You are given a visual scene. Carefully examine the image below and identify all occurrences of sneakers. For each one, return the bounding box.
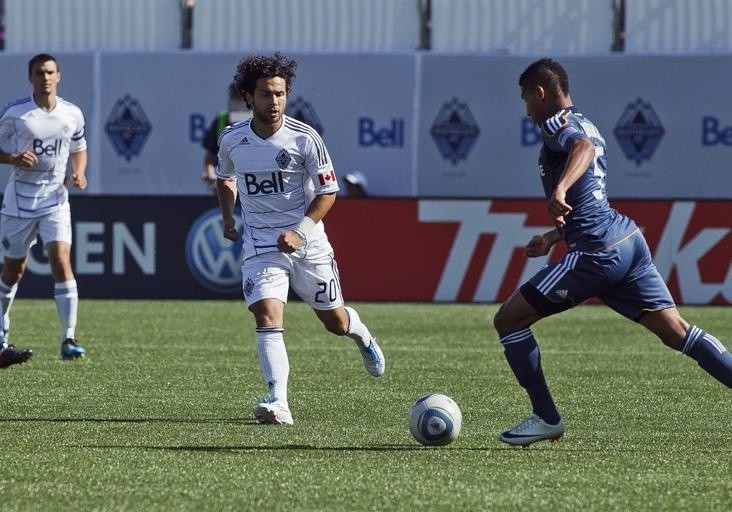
[61,338,85,360]
[359,333,385,377]
[499,414,564,448]
[254,401,293,425]
[0,342,33,368]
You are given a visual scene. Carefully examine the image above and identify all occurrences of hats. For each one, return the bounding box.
[345,171,368,190]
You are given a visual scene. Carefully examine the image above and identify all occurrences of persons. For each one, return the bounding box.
[203,83,253,189]
[0,301,33,368]
[0,53,87,358]
[214,53,386,425]
[494,59,732,445]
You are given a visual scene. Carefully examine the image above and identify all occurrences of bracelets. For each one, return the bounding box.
[292,215,316,239]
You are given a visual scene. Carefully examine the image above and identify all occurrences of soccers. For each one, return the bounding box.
[407,393,462,446]
